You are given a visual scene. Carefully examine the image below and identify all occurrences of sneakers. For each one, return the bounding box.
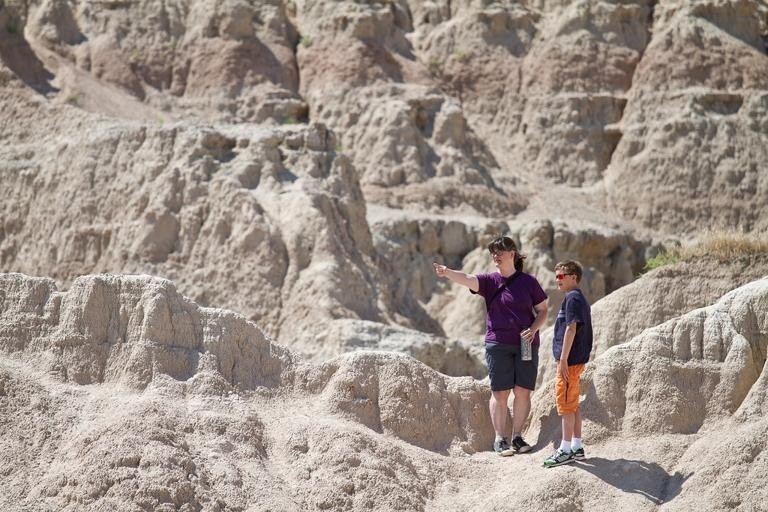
[543,447,575,468]
[511,435,532,454]
[493,438,514,457]
[571,447,585,461]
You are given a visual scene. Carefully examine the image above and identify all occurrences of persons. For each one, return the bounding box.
[433,236,548,456]
[543,260,593,467]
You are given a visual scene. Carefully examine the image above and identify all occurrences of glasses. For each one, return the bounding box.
[555,273,572,279]
[490,250,513,257]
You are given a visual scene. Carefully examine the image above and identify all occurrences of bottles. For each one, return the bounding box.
[520,327,532,361]
